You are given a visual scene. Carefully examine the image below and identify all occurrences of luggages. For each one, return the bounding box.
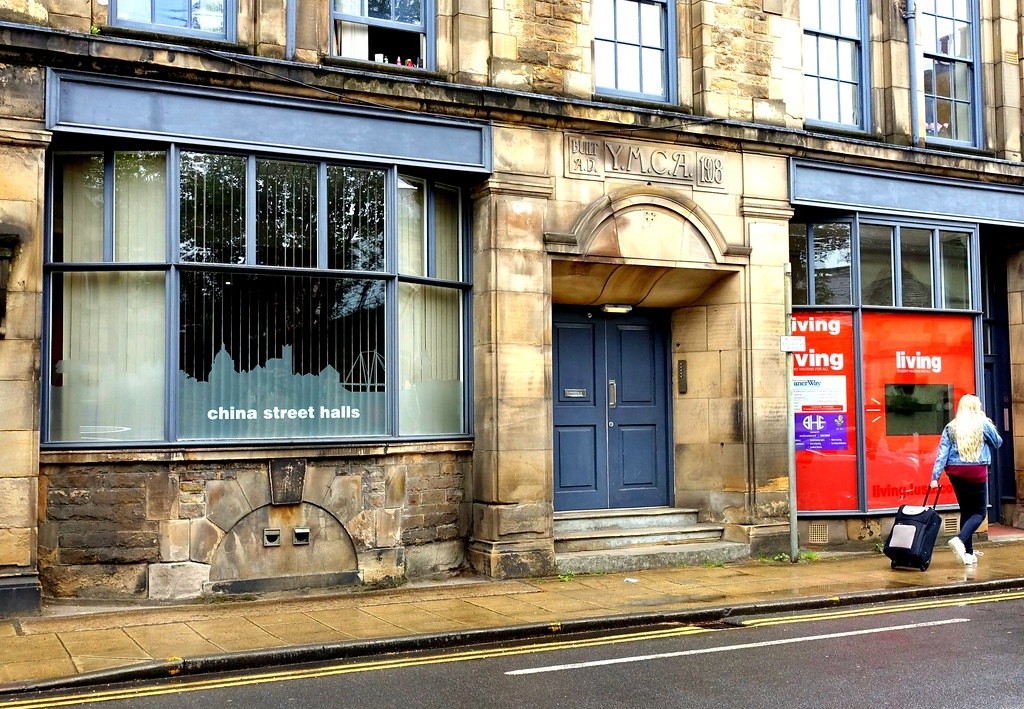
[883,483,942,572]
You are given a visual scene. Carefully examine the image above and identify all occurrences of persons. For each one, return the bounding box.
[928,393,1003,566]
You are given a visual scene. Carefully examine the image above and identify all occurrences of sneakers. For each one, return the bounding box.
[948,536,985,565]
